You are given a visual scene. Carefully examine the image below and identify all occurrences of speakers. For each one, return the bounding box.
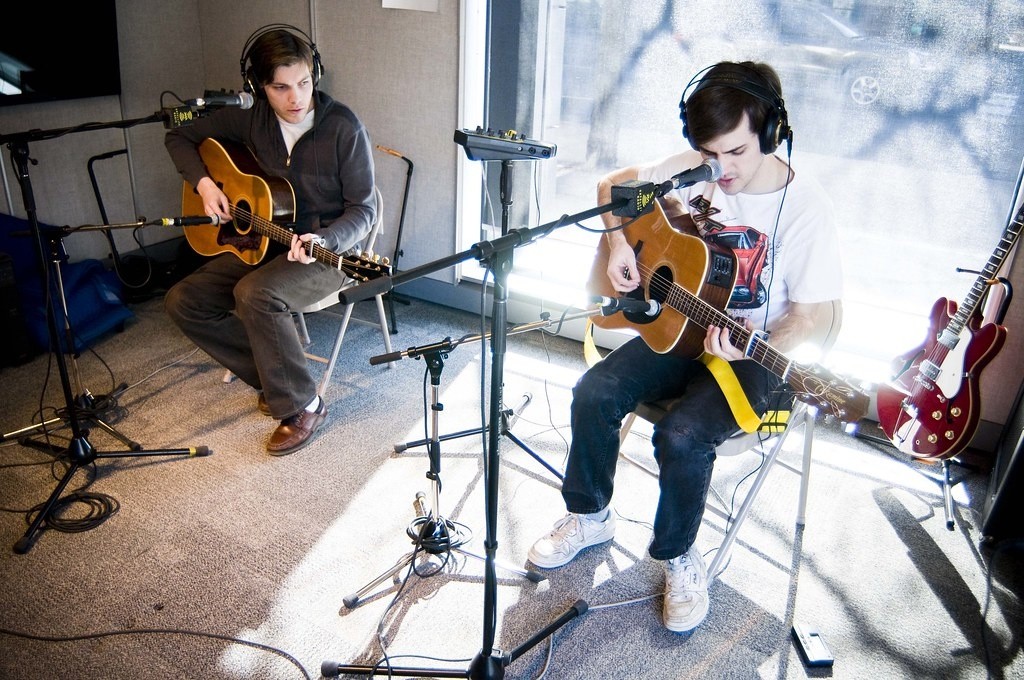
[979,377,1024,539]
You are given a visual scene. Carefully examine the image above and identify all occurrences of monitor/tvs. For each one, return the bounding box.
[0,0,121,107]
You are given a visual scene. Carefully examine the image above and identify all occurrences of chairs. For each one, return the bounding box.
[221,185,396,399]
[623,296,842,586]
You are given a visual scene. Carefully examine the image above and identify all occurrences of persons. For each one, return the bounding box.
[163,30,376,455]
[527,61,845,631]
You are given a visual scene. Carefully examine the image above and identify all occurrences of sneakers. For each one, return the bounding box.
[658,542,709,631]
[528,507,617,569]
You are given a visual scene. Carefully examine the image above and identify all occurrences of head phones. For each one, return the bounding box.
[239,22,325,101]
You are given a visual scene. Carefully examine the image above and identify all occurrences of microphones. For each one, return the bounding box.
[183,91,254,110]
[591,294,662,317]
[154,212,221,228]
[653,158,724,198]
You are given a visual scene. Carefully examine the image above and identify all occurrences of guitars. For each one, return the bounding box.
[584,193,870,424]
[876,204,1023,462]
[180,136,392,284]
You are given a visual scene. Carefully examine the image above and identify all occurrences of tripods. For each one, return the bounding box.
[847,156,1024,528]
[0,145,208,554]
[321,160,590,680]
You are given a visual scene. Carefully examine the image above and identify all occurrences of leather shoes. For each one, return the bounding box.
[257,391,271,416]
[265,394,330,457]
